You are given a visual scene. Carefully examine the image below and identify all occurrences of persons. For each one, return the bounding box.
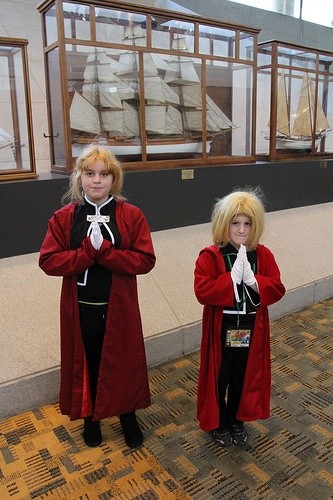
[38,148,156,448]
[193,191,286,445]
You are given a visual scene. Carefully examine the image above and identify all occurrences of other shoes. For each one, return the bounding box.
[209,427,232,446]
[231,419,248,446]
[83,415,102,447]
[120,412,145,448]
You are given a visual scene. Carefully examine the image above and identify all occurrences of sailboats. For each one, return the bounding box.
[264,67,332,154]
[70,24,239,161]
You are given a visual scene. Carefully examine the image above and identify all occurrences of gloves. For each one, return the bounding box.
[230,243,243,285]
[90,222,98,250]
[243,245,256,286]
[95,221,104,251]
